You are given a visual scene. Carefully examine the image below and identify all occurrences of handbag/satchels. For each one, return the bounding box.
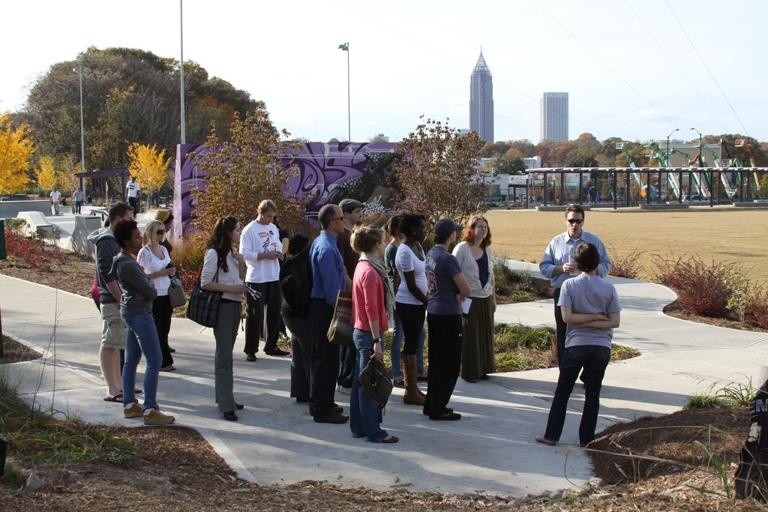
[327,288,354,346]
[359,357,392,407]
[167,263,187,308]
[186,248,223,327]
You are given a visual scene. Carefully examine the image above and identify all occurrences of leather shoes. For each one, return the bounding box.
[224,412,238,422]
[444,407,453,412]
[536,436,556,445]
[265,347,290,355]
[236,402,243,409]
[313,413,350,423]
[308,406,343,415]
[246,353,256,361]
[369,434,400,444]
[429,411,461,420]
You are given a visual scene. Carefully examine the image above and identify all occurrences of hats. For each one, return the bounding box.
[339,198,366,214]
[433,218,464,244]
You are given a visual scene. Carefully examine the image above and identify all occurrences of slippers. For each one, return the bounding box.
[121,387,142,394]
[104,394,137,403]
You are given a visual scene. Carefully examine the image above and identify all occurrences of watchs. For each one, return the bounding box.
[373,337,382,343]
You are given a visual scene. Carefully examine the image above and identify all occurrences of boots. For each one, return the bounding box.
[402,354,426,405]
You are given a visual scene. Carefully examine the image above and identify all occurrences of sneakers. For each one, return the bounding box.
[143,409,177,425]
[340,386,351,393]
[124,402,143,418]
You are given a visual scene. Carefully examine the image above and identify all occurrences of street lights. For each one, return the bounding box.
[333,41,350,141]
[73,64,86,198]
[666,129,680,202]
[688,125,702,202]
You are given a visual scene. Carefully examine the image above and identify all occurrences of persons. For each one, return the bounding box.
[336,199,366,392]
[539,204,612,388]
[50,187,62,215]
[87,204,143,403]
[395,213,429,405]
[239,199,290,361]
[261,216,288,341]
[125,176,141,219]
[351,226,398,444]
[143,209,175,352]
[73,188,84,214]
[381,215,427,389]
[280,234,310,403]
[423,219,471,421]
[306,205,349,425]
[536,244,622,447]
[107,218,175,425]
[136,220,175,371]
[201,216,247,421]
[452,216,496,383]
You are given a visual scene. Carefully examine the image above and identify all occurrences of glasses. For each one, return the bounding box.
[327,215,344,222]
[568,218,583,224]
[156,229,165,236]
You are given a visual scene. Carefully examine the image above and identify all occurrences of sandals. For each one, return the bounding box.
[158,366,176,371]
